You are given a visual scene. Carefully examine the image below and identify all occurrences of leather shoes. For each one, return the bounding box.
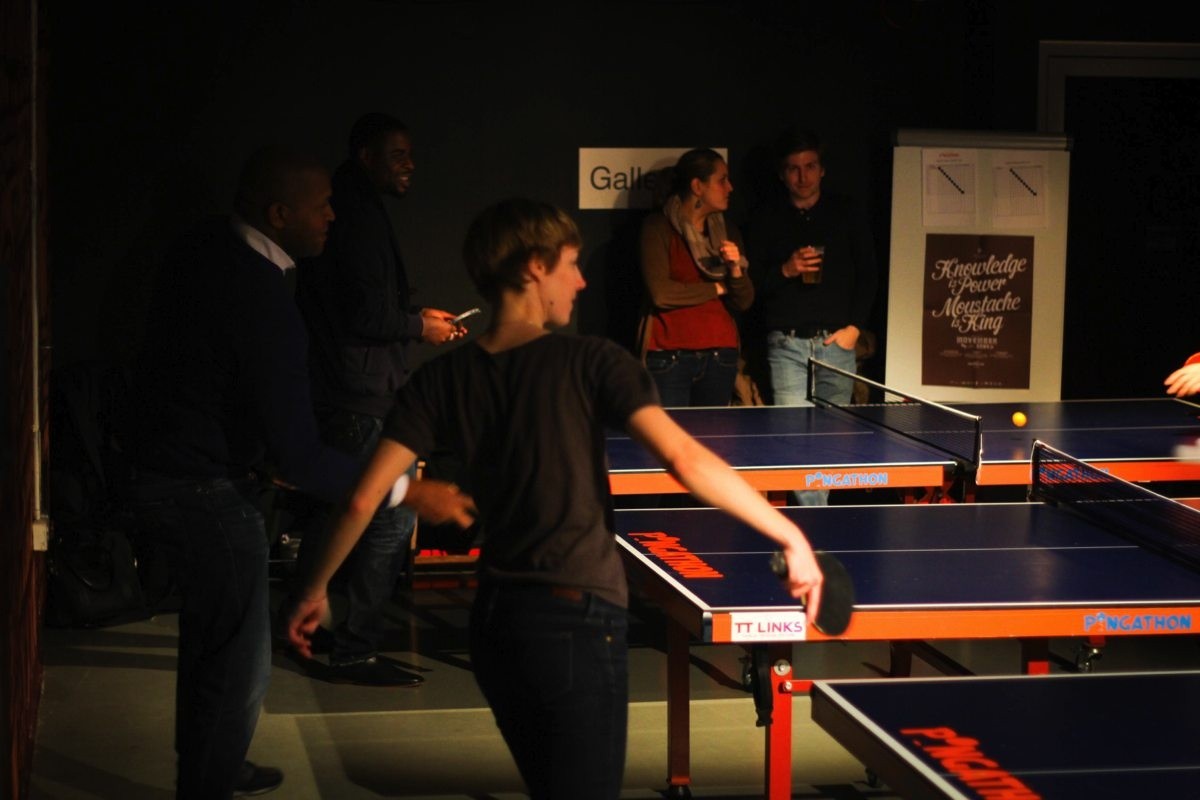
[305,655,423,686]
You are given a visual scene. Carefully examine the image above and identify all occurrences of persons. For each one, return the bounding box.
[294,111,468,686]
[635,148,755,405]
[133,144,480,800]
[744,131,877,406]
[1164,351,1200,398]
[289,198,826,800]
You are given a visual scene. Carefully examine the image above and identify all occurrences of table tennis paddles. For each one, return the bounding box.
[773,551,855,635]
[1183,349,1200,374]
[439,305,483,333]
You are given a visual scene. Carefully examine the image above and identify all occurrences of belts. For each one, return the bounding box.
[783,322,823,339]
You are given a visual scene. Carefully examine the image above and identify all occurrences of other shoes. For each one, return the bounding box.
[227,756,283,797]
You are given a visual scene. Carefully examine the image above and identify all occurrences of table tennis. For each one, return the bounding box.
[1012,411,1028,428]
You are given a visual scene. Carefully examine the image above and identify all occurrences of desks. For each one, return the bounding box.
[602,398,1200,800]
